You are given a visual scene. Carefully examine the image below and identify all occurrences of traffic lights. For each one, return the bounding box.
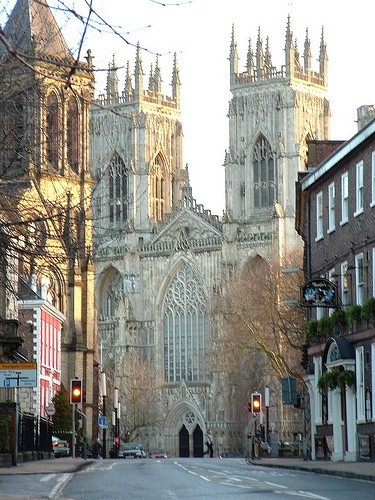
[247,401,252,413]
[250,394,262,414]
[70,379,82,404]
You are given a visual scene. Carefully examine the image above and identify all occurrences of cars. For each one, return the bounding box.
[120,443,147,460]
[51,436,69,457]
[149,449,167,459]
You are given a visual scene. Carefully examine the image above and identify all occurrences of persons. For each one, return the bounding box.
[205,429,214,458]
[253,434,261,458]
[94,437,103,459]
[76,437,86,459]
